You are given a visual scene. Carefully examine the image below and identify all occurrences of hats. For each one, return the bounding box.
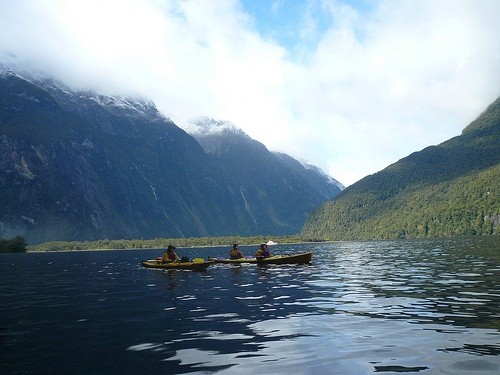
[260,243,267,248]
[166,245,176,250]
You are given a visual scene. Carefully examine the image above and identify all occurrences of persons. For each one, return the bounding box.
[255,244,273,258]
[229,244,245,259]
[162,245,181,264]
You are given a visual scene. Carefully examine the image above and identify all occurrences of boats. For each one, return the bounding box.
[207,252,312,266]
[142,257,216,272]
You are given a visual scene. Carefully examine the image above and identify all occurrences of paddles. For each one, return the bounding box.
[141,257,204,263]
